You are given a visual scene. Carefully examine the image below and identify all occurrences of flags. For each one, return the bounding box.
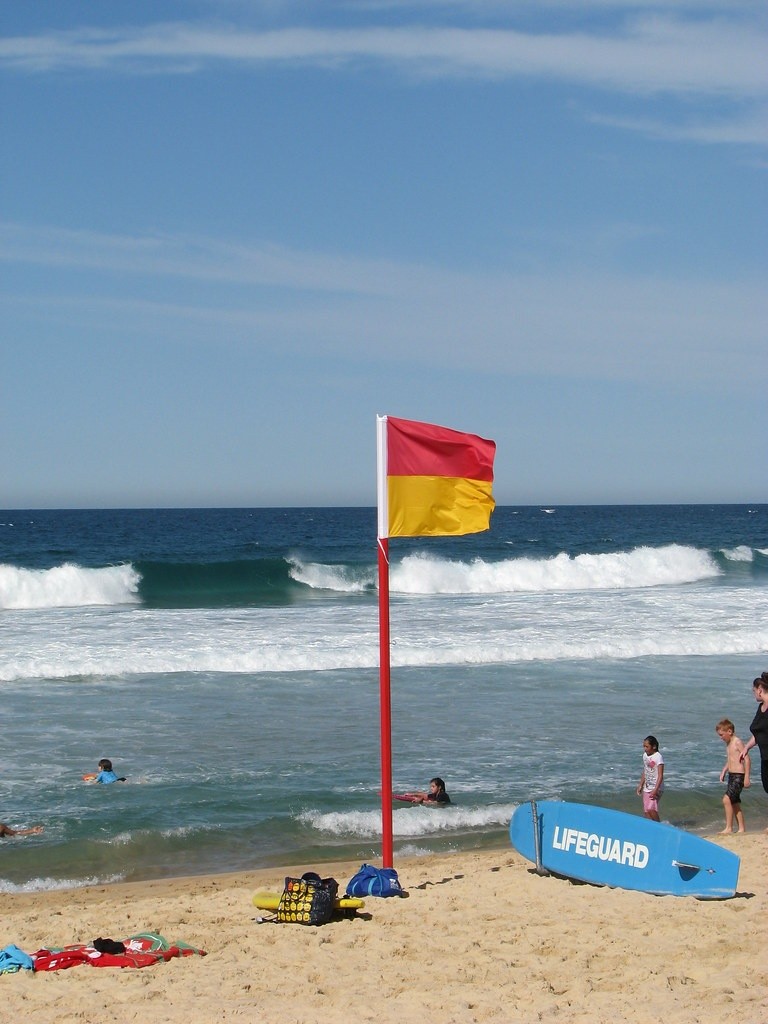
[377,415,496,539]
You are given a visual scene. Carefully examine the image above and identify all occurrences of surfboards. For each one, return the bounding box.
[508,798,743,902]
[376,791,437,805]
[83,772,100,783]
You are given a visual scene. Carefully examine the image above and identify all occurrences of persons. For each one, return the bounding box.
[739,671,768,833]
[716,719,752,834]
[404,778,451,806]
[637,736,665,823]
[92,759,119,785]
[0,822,44,839]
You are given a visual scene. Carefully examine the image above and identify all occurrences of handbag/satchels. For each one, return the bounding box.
[276,877,340,927]
[345,863,402,898]
